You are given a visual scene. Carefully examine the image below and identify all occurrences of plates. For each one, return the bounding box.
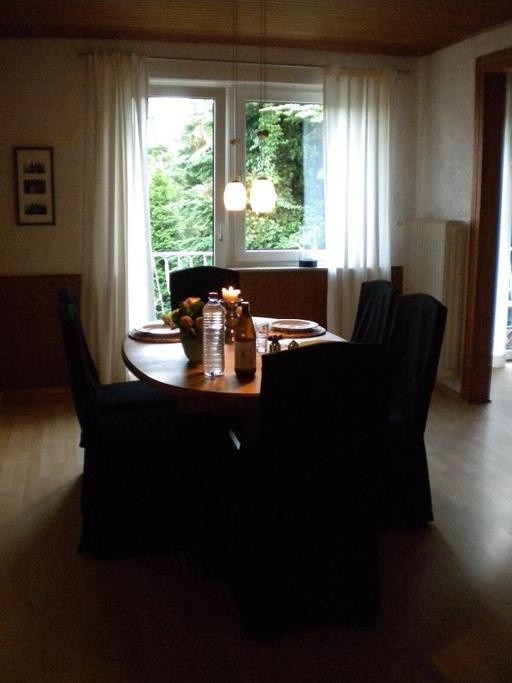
[269,318,319,332]
[134,320,180,336]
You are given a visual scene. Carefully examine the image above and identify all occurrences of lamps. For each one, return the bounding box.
[222,0,277,215]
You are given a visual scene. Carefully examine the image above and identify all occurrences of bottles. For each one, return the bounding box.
[202,291,225,378]
[234,301,257,376]
[222,302,239,345]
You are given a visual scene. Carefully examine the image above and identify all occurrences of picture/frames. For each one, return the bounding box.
[14,144,56,226]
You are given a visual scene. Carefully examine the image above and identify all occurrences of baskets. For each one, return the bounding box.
[180,329,203,363]
